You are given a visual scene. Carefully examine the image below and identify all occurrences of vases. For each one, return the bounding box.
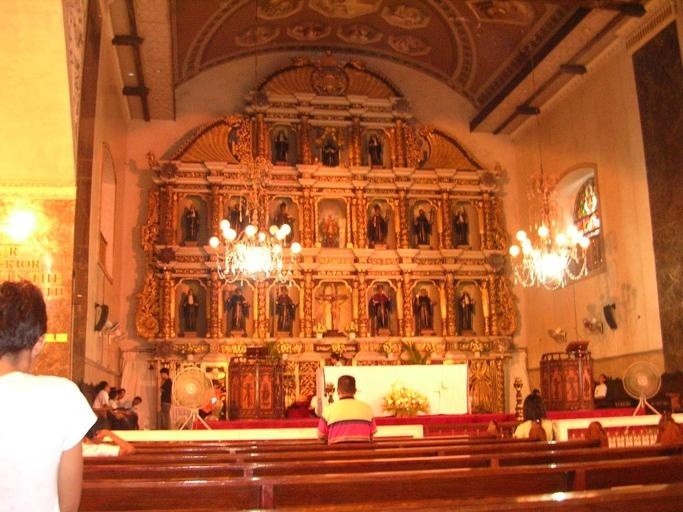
[395,411,417,418]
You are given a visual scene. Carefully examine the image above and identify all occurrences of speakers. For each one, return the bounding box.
[96,304,108,332]
[602,304,618,330]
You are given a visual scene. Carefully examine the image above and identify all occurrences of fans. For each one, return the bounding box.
[622,360,663,416]
[171,367,214,430]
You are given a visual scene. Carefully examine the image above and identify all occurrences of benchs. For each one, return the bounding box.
[75,422,682,512]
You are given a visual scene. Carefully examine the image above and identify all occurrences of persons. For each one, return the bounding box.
[513,393,554,441]
[273,129,288,162]
[159,368,173,431]
[84,377,143,457]
[181,201,469,247]
[182,285,476,331]
[369,135,382,166]
[318,375,377,445]
[322,135,338,167]
[0,278,98,512]
[594,374,608,400]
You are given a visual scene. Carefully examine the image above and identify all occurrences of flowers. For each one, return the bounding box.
[383,386,430,415]
